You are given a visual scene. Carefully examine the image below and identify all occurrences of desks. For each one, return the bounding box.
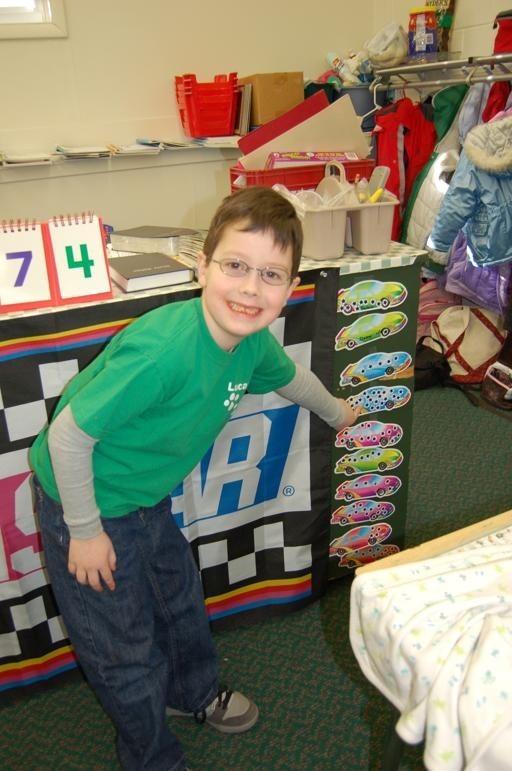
[0,229,429,584]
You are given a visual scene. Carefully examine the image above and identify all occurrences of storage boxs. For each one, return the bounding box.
[238,71,305,128]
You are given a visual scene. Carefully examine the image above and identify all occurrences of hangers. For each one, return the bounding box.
[359,79,412,127]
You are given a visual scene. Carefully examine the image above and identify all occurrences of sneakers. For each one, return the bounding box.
[166,688,259,733]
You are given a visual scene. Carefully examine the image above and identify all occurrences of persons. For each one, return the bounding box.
[28,187,362,771]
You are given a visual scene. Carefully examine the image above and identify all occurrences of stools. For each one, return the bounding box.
[175,69,238,139]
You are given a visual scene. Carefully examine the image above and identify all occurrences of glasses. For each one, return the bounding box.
[207,255,295,286]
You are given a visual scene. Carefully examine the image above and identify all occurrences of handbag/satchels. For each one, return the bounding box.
[414,305,512,411]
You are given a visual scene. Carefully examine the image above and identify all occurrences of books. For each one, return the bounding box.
[107,251,195,293]
[0,134,242,168]
